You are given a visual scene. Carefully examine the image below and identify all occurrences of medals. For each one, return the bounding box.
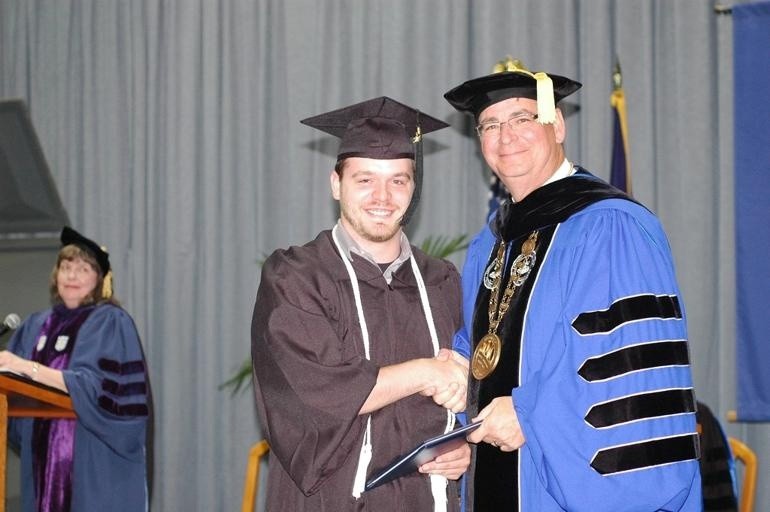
[471,332,502,381]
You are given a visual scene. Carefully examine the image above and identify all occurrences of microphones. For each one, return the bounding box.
[0,311,21,343]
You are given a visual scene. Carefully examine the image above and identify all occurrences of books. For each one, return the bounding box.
[361,418,483,491]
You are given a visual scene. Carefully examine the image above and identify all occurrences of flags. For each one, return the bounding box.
[606,87,633,197]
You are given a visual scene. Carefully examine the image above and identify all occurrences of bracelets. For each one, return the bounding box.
[29,360,40,382]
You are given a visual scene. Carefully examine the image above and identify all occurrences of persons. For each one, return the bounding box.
[0,227,152,510]
[248,97,471,511]
[418,64,705,511]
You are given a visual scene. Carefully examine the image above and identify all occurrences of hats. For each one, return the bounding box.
[444,58,583,124]
[60,226,111,278]
[300,95,451,159]
